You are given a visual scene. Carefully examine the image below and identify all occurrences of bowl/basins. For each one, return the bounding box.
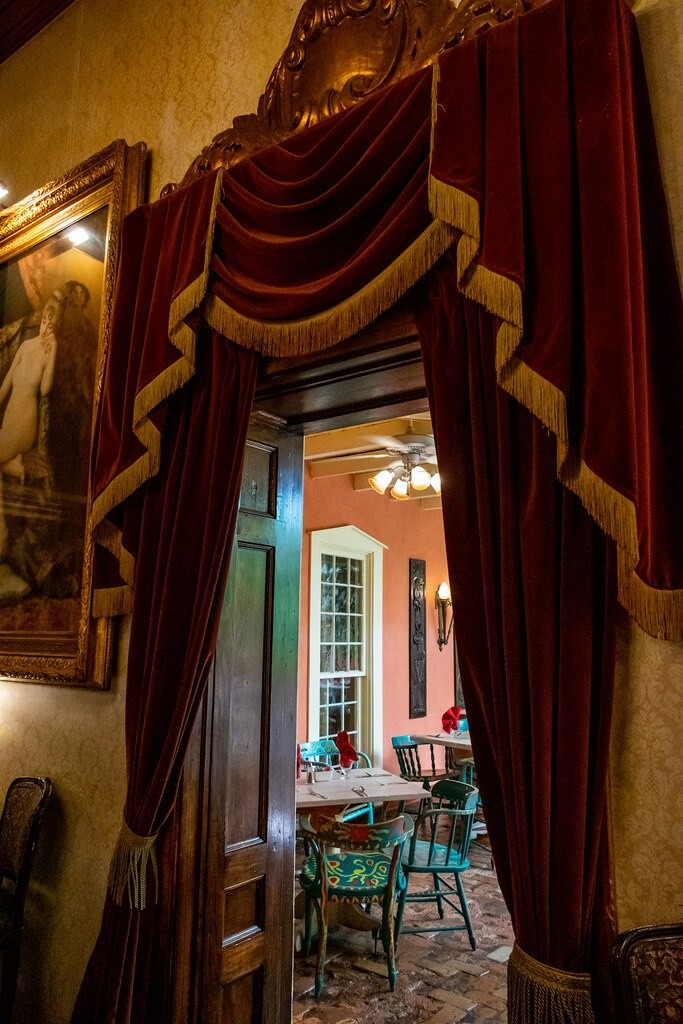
[313,766,334,782]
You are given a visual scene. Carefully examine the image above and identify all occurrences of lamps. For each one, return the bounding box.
[368,419,440,500]
[434,582,453,652]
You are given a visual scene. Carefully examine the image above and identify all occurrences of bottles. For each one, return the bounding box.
[306,761,314,784]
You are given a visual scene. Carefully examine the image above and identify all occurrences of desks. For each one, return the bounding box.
[296,766,432,809]
[409,732,472,750]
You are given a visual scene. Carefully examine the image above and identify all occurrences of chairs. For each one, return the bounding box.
[459,759,496,867]
[451,719,480,802]
[291,815,416,997]
[612,919,683,1024]
[0,776,52,1022]
[305,751,374,825]
[392,736,443,821]
[383,777,479,951]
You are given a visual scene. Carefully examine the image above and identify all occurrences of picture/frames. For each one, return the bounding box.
[1,135,148,693]
